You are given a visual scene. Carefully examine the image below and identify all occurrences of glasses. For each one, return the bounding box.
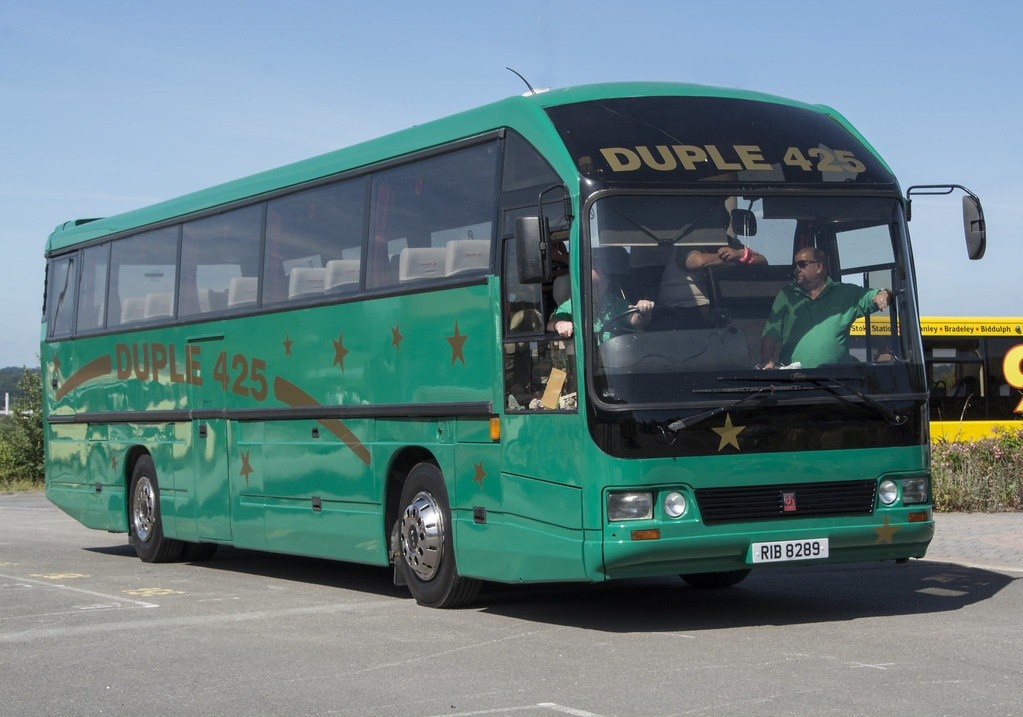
[791,260,818,269]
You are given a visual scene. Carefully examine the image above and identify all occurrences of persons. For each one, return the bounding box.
[758,247,891,369]
[658,208,769,306]
[546,264,655,371]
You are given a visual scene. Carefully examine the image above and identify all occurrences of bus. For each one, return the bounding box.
[42,81,987,608]
[848,315,1023,444]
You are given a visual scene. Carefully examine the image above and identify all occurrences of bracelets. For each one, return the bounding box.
[553,320,561,331]
[739,246,753,263]
[761,359,773,362]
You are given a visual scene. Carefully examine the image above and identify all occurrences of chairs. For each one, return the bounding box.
[69,218,787,357]
[930,374,1023,421]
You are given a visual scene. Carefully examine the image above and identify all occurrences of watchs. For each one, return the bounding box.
[877,288,889,295]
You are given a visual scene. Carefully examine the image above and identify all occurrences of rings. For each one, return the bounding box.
[880,298,883,301]
